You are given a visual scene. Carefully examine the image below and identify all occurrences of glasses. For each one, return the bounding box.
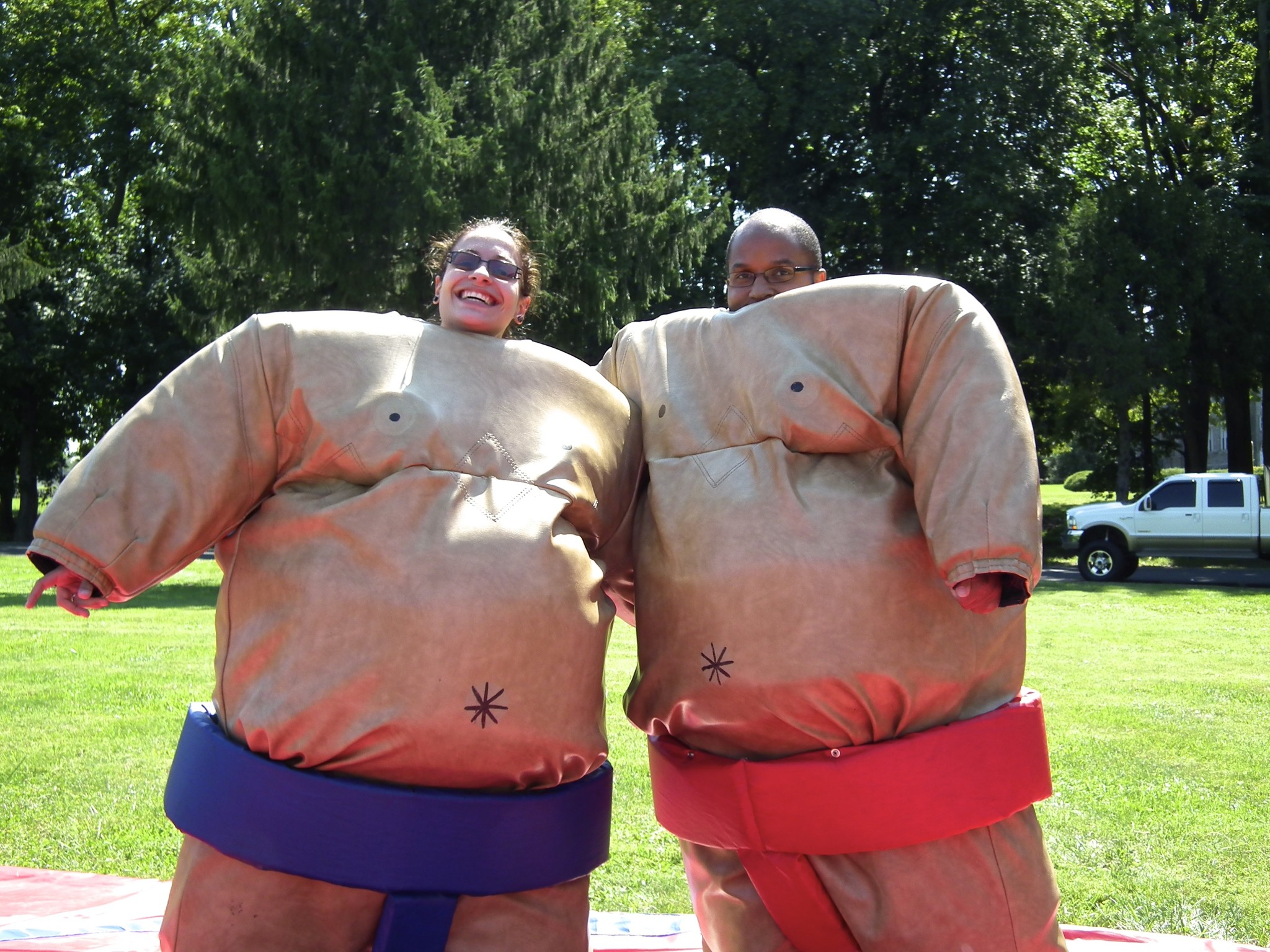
[725,264,821,288]
[441,250,524,288]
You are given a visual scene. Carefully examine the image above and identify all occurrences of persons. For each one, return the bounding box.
[25,218,644,952]
[592,207,1069,952]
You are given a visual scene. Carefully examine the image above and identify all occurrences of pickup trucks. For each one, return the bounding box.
[1066,474,1269,585]
